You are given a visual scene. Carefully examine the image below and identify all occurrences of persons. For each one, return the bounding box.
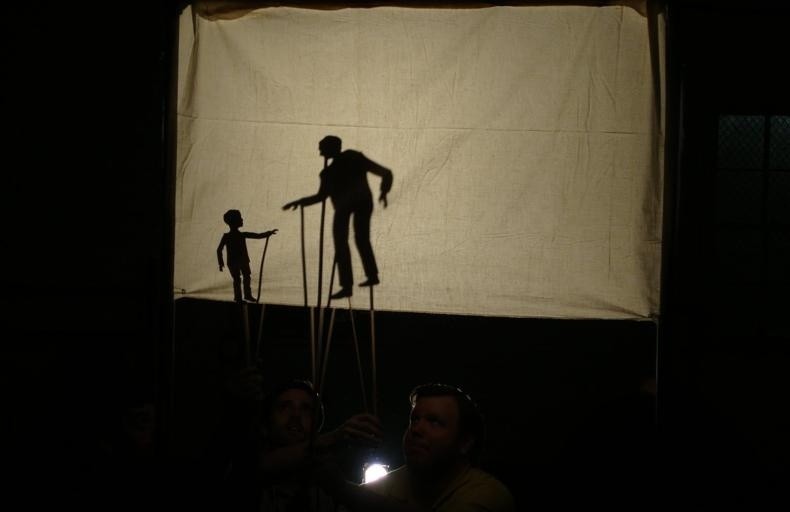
[224,374,515,512]
